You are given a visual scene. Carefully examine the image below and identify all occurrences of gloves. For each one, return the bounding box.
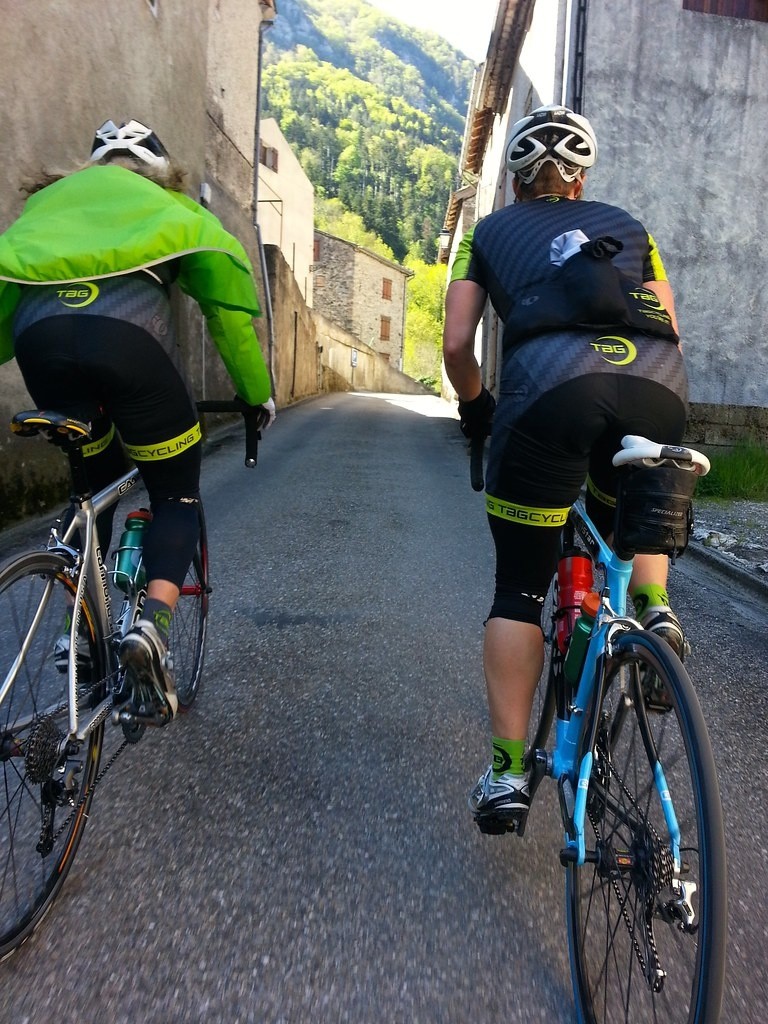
[457,384,496,439]
[233,392,277,433]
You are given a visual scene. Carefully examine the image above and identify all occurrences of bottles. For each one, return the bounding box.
[113,509,154,594]
[560,593,602,687]
[556,546,594,655]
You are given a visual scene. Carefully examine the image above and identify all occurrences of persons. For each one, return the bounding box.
[0,118,277,722]
[443,104,689,816]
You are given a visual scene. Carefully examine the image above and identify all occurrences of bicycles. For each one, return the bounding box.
[1,393,271,971]
[458,424,729,1024]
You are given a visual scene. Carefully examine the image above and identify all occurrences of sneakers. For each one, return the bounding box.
[634,606,685,713]
[467,763,531,814]
[116,618,179,728]
[54,634,93,684]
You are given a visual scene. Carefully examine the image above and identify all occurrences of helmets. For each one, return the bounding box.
[503,103,599,185]
[89,119,172,175]
